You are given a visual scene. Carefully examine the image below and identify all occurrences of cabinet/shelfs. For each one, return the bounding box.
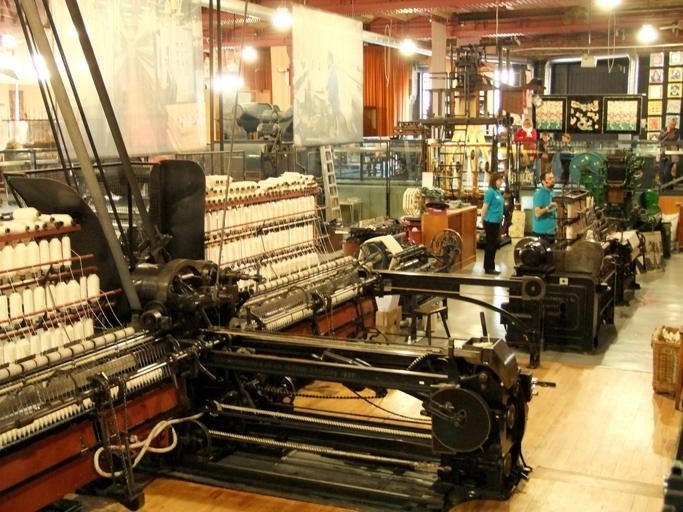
[422,206,477,272]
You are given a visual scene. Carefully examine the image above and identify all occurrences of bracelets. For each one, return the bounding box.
[545,205,550,212]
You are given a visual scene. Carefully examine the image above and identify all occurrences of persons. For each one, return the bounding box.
[514,118,538,173]
[539,132,557,173]
[480,173,512,275]
[559,132,573,182]
[659,117,680,190]
[531,171,559,242]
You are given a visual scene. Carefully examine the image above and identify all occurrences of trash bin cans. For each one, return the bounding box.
[651,325,681,394]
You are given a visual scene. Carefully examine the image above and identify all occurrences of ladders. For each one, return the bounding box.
[320,145,342,224]
